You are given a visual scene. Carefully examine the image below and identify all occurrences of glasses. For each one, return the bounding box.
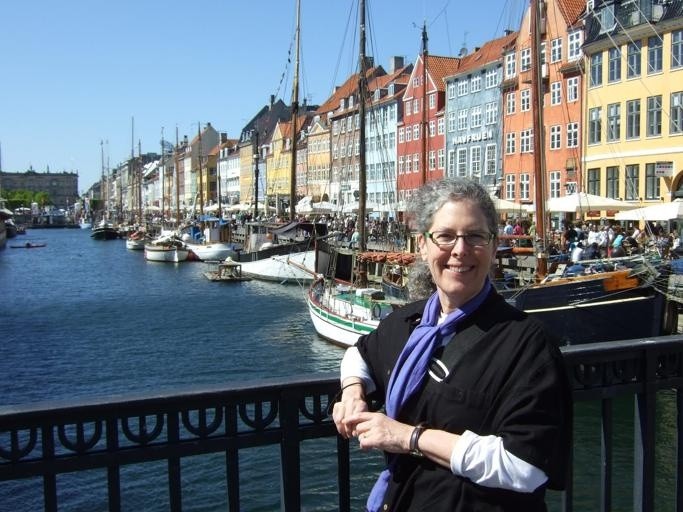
[423,231,494,246]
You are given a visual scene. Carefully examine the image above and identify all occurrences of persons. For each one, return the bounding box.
[332,177,574,512]
[497,218,683,278]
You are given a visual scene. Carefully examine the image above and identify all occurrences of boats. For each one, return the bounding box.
[201,256,254,282]
[1,208,79,248]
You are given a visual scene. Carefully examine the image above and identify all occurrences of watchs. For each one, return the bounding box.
[407,421,433,457]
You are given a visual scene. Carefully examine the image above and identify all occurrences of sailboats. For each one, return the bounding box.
[117,0,340,286]
[309,1,669,382]
[90,140,119,240]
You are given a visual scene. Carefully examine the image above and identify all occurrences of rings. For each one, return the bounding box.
[362,433,368,439]
